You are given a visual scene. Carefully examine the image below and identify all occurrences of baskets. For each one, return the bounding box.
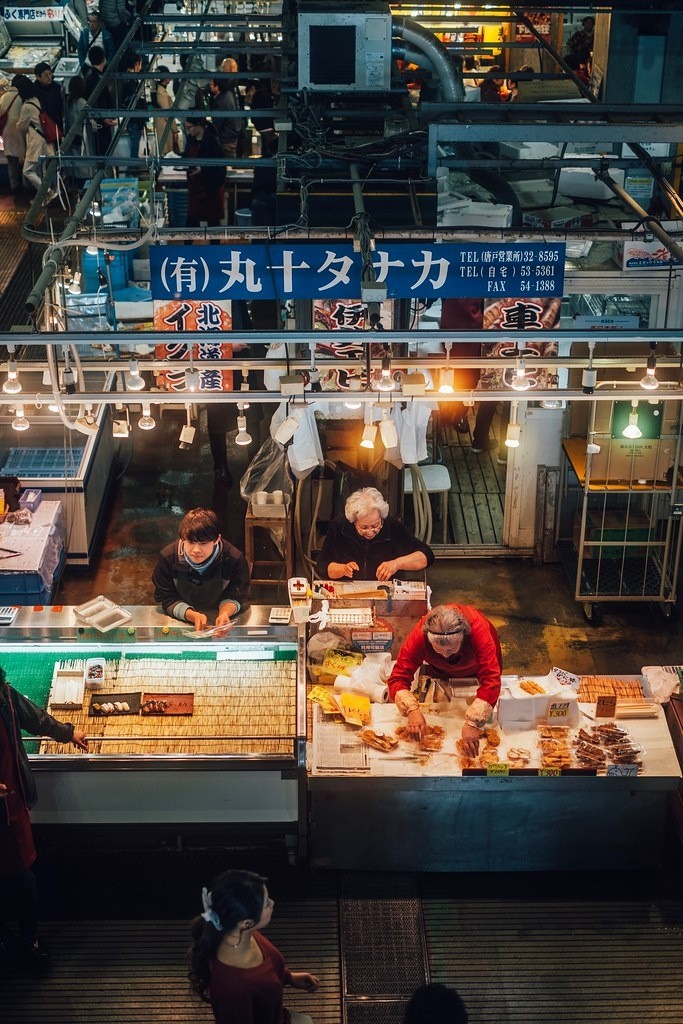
[18,488,42,513]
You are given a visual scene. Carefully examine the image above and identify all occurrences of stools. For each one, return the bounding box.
[244,504,293,602]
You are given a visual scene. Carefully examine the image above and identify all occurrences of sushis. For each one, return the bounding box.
[93,702,130,712]
[142,699,167,713]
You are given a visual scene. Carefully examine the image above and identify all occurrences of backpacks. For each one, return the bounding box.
[23,101,64,144]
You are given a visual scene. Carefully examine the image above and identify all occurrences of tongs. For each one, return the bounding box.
[0,548,22,560]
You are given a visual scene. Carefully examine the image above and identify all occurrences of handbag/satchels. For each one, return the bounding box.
[0,795,11,829]
[238,126,253,159]
[171,131,180,155]
[0,112,8,138]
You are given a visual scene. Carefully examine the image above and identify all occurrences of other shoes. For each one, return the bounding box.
[214,469,233,486]
[453,413,470,433]
[0,924,49,960]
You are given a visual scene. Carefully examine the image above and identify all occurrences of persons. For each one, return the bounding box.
[566,16,596,55]
[205,299,264,486]
[0,668,89,964]
[471,401,511,464]
[184,869,320,1024]
[321,487,435,582]
[387,604,503,758]
[151,507,250,638]
[0,0,264,245]
[400,983,469,1024]
[450,54,593,102]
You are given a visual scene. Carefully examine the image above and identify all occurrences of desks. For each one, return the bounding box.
[304,675,683,887]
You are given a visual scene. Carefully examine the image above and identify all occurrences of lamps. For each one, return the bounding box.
[440,341,455,393]
[639,345,659,389]
[235,375,253,446]
[274,405,305,445]
[127,357,145,390]
[110,402,156,439]
[344,356,398,449]
[581,347,596,395]
[68,270,80,295]
[48,379,99,436]
[623,396,642,438]
[177,347,200,452]
[85,239,99,257]
[1,340,30,432]
[506,342,530,446]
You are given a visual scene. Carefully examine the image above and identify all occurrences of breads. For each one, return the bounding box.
[520,680,545,695]
[538,724,643,768]
[354,724,530,766]
[575,677,645,703]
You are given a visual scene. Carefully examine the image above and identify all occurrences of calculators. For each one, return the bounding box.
[0,607,18,624]
[269,608,292,624]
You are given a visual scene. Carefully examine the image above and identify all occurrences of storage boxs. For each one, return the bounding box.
[0,500,67,607]
[85,656,105,690]
[251,490,290,518]
[571,509,655,560]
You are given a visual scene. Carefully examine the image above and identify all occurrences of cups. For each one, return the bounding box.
[272,490,282,505]
[256,491,267,505]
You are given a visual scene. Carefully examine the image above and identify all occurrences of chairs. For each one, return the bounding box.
[401,464,451,545]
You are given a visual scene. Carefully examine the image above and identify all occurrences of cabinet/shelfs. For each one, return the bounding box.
[552,341,683,629]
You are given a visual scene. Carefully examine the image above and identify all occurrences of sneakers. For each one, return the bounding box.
[472,438,500,452]
[496,451,508,465]
[41,189,57,206]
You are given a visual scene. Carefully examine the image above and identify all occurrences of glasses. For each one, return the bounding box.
[355,517,384,535]
